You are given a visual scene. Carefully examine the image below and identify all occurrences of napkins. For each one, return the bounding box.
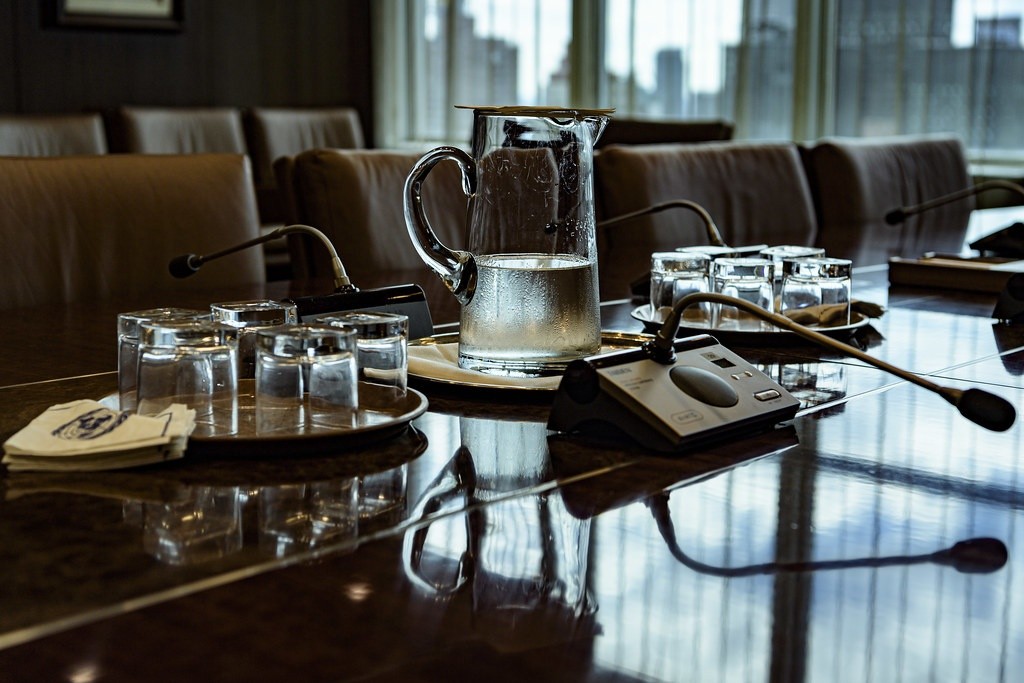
[1,397,198,471]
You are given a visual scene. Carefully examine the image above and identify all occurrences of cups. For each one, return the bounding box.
[649,245,852,332]
[745,359,847,410]
[120,465,412,566]
[115,300,409,438]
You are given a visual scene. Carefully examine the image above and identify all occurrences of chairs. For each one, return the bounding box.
[1,103,977,392]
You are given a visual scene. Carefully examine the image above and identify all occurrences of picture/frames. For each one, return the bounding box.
[38,0,189,35]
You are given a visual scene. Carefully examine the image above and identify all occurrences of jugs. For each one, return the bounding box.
[400,411,600,630]
[401,107,608,376]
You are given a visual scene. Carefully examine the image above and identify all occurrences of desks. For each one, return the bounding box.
[0,185,1024,683]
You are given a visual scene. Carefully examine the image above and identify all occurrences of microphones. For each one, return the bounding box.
[169,225,434,343]
[885,179,1024,226]
[547,293,1016,459]
[547,433,1009,582]
[544,198,728,251]
